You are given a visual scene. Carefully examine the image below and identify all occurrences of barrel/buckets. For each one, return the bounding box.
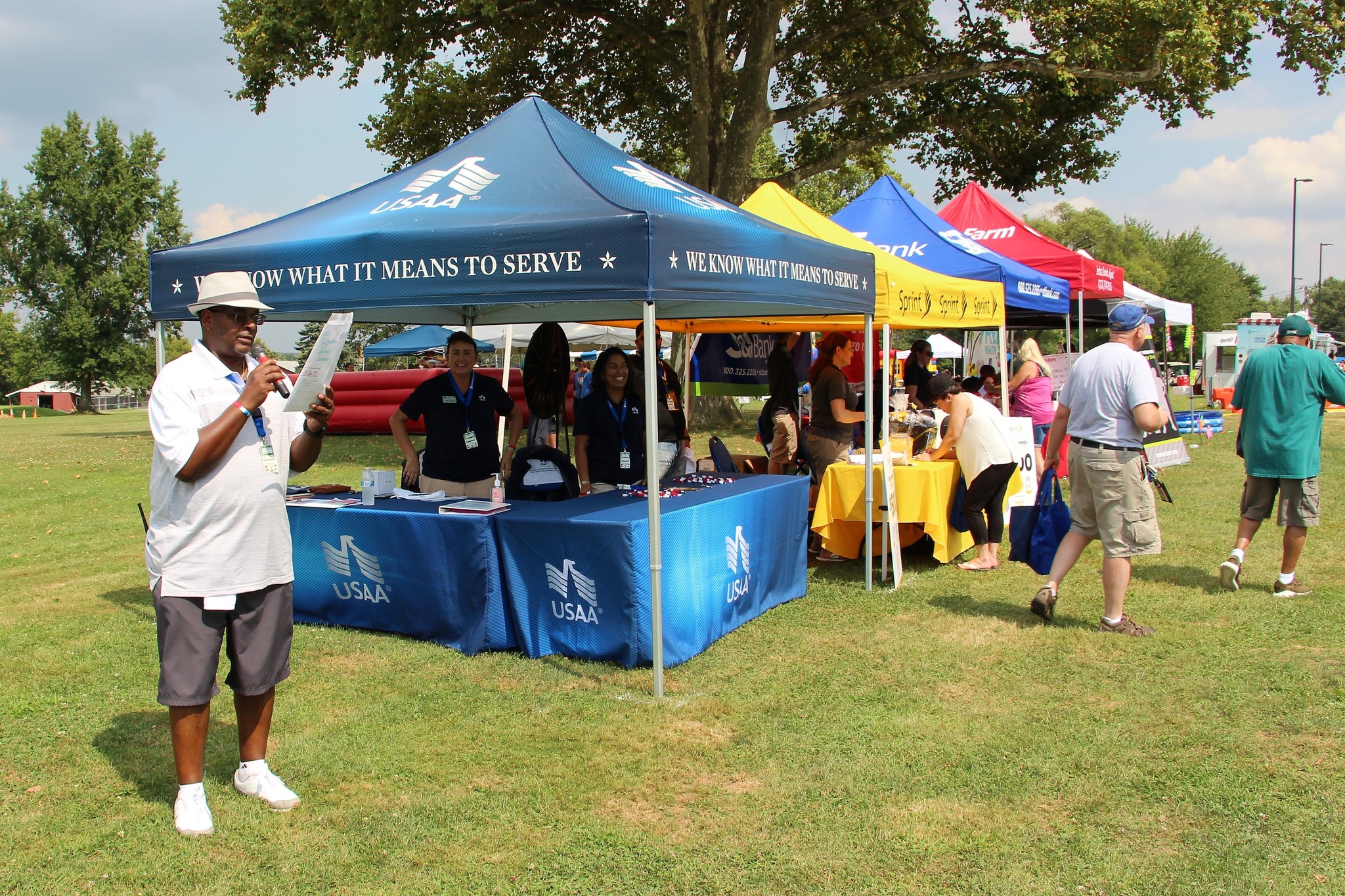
[1176,374,1189,386]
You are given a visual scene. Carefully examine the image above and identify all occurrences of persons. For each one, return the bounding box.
[916,371,1018,571]
[345,363,354,372]
[622,320,693,484]
[145,267,338,841]
[390,332,523,498]
[414,346,450,368]
[1221,315,1345,596]
[819,175,1071,538]
[519,345,595,452]
[761,332,1061,490]
[166,93,875,693]
[1028,304,1170,642]
[574,346,662,498]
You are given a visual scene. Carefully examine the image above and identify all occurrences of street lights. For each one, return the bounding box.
[1290,177,1313,313]
[1293,277,1303,312]
[1318,242,1334,333]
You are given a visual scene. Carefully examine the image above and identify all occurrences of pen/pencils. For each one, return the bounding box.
[702,484,712,489]
[617,484,644,491]
[680,490,686,493]
[409,493,432,496]
[930,443,933,449]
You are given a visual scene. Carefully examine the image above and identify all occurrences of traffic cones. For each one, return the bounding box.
[21,410,26,418]
[0,409,4,415]
[33,408,37,418]
[10,408,14,418]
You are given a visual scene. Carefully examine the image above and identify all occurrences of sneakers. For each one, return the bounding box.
[1273,577,1313,597]
[1096,612,1156,638]
[1030,585,1058,620]
[234,762,301,812]
[1219,555,1240,591]
[174,785,215,837]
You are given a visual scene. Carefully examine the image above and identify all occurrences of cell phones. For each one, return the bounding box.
[376,493,395,500]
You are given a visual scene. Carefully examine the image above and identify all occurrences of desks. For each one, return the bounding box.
[286,449,1025,672]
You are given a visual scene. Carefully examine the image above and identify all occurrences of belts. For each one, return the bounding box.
[1069,435,1141,452]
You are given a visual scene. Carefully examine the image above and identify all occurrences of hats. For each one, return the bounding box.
[926,373,955,400]
[1108,303,1157,330]
[187,271,275,316]
[1278,315,1311,336]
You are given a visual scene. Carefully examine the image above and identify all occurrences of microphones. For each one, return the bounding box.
[250,343,290,399]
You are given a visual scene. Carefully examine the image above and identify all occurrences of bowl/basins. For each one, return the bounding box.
[878,437,914,466]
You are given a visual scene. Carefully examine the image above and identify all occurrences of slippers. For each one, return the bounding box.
[808,547,821,554]
[955,562,1000,572]
[816,553,849,562]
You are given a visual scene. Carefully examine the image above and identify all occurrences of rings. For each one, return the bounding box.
[322,407,328,415]
[406,473,410,475]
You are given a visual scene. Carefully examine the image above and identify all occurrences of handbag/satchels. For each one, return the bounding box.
[1026,468,1072,575]
[1235,422,1244,458]
[1008,468,1049,571]
[948,475,970,532]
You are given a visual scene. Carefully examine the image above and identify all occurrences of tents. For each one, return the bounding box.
[892,333,975,377]
[360,322,497,374]
[933,184,1197,405]
[569,181,1009,592]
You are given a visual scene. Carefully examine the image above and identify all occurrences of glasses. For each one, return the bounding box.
[213,310,266,325]
[922,351,933,357]
[845,348,852,352]
[637,333,662,341]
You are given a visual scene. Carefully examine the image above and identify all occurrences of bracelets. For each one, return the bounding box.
[579,490,591,495]
[1045,451,1060,459]
[231,400,252,419]
[303,418,328,438]
[505,443,516,452]
[682,436,690,441]
[579,480,591,484]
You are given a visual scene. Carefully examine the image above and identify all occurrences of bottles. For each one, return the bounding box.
[362,467,375,506]
[889,432,911,444]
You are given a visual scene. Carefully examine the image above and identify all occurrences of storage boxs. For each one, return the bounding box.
[696,453,769,475]
[1176,375,1189,386]
[1172,386,1191,395]
[361,470,396,495]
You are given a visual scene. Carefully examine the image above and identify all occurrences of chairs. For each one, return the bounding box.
[400,449,425,494]
[506,445,580,502]
[709,414,818,486]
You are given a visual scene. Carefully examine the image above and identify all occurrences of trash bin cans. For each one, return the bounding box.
[1176,374,1189,386]
